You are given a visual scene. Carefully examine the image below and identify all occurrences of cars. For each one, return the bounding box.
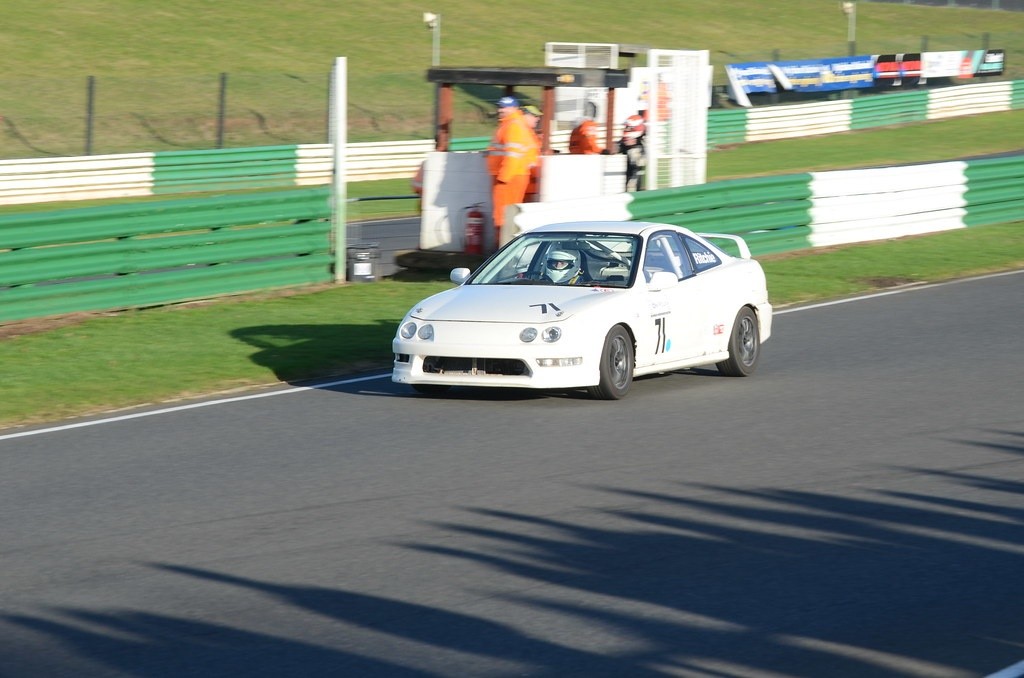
[390,222,773,401]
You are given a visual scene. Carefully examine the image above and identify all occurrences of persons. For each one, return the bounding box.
[569,101,611,154]
[487,97,536,242]
[520,103,544,202]
[542,242,585,285]
[622,110,647,191]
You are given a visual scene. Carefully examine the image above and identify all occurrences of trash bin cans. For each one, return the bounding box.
[347,243,382,282]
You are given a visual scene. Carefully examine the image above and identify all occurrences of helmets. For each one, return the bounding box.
[545,245,580,284]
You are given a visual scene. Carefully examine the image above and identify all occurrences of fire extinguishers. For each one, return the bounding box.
[463,201,487,255]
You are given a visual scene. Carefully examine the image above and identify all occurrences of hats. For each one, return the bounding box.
[495,96,520,108]
[522,105,544,117]
